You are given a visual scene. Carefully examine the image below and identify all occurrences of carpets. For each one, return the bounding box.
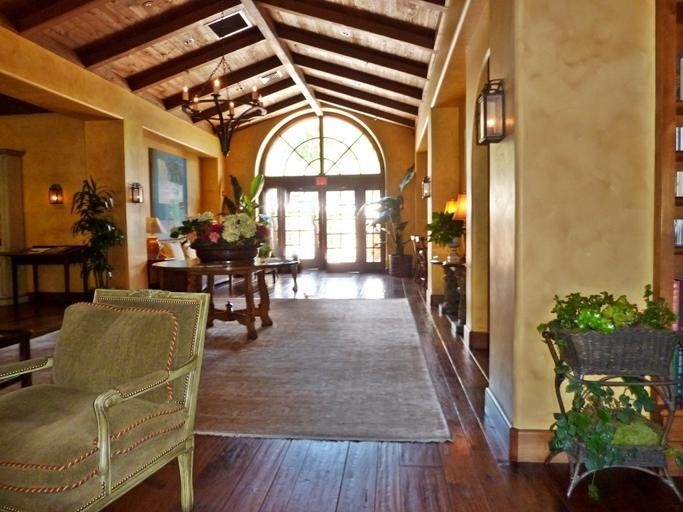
[1,298,454,445]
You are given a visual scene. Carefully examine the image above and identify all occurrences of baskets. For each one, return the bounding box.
[574,438,668,468]
[554,328,680,377]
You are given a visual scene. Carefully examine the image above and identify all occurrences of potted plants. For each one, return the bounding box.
[356,196,414,277]
[535,285,683,501]
[424,210,464,260]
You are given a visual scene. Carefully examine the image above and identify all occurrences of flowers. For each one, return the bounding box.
[170,211,271,250]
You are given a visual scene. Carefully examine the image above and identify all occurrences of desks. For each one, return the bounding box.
[150,259,285,340]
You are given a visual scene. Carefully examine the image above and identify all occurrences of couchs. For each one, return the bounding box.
[157,238,234,291]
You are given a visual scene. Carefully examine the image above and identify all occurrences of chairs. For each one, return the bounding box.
[0,287,212,512]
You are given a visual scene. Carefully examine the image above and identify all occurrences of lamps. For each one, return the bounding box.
[49,184,64,204]
[180,1,268,158]
[146,216,162,260]
[443,191,466,229]
[129,183,144,203]
[422,176,431,199]
[476,78,506,145]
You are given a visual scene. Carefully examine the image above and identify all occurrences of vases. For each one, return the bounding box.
[196,247,260,266]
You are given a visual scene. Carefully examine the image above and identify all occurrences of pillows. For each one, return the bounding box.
[51,301,179,404]
[161,241,197,261]
